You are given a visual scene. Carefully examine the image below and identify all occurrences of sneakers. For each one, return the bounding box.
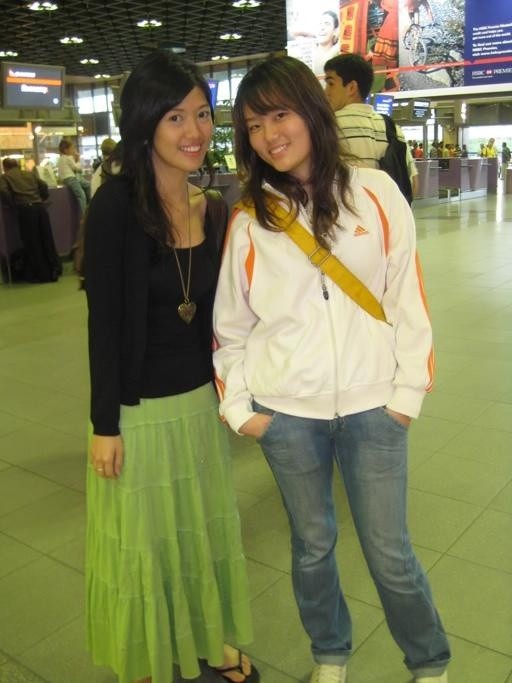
[309,664,346,683]
[416,672,447,683]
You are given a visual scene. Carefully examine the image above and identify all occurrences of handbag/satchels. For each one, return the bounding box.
[379,141,413,204]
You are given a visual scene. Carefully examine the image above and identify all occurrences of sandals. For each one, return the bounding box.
[216,647,260,683]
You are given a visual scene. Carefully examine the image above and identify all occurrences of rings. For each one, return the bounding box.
[97,467,105,472]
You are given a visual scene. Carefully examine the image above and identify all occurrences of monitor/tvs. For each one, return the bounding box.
[373,94,394,118]
[410,99,430,122]
[0,62,66,112]
[206,79,219,111]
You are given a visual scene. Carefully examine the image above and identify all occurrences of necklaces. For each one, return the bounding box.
[159,181,198,326]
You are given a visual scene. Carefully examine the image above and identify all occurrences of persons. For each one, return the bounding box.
[407,136,511,179]
[0,156,64,283]
[81,47,261,682]
[89,135,122,200]
[323,51,420,207]
[209,54,453,683]
[57,138,92,215]
[301,10,340,65]
